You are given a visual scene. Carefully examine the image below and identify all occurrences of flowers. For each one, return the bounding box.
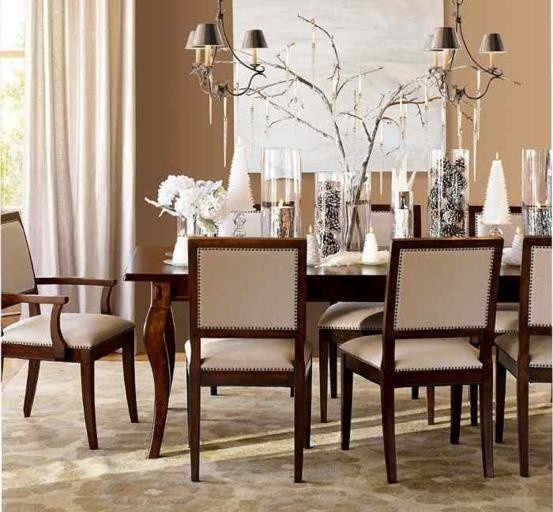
[140,174,230,238]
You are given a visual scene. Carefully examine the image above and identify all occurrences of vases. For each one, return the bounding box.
[339,170,372,255]
[171,214,195,267]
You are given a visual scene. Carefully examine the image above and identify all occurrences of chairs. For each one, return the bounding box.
[0,210,138,450]
[316,204,421,424]
[495,234,552,478]
[184,236,313,483]
[337,236,505,484]
[467,204,552,427]
